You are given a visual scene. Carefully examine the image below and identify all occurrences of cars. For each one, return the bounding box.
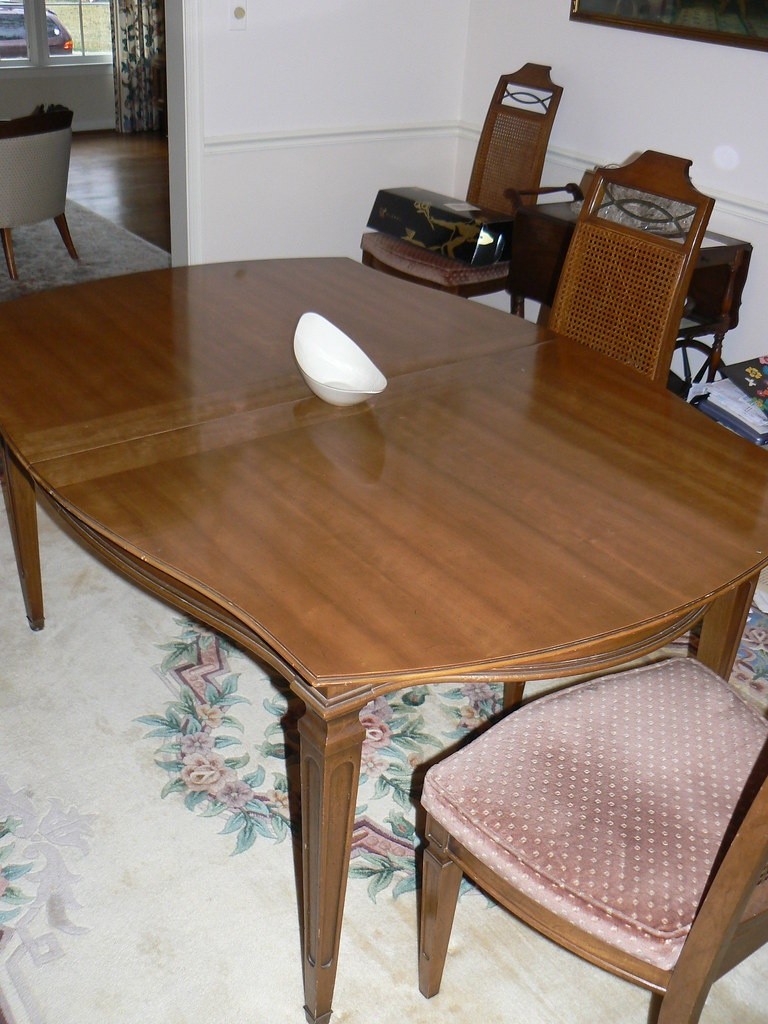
[0,2,74,59]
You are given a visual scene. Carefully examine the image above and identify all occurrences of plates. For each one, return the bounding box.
[291,311,387,406]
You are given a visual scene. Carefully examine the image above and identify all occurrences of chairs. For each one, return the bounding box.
[417,656,767,1023]
[361,62,564,319]
[505,150,715,714]
[0,109,79,282]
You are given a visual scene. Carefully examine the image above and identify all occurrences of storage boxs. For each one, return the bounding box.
[366,186,517,266]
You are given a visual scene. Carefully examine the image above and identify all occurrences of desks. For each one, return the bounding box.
[0,253,768,1023]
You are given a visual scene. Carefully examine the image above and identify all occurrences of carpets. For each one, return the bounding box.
[0,198,171,299]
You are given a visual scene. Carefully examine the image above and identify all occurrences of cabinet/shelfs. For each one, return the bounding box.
[507,200,753,383]
